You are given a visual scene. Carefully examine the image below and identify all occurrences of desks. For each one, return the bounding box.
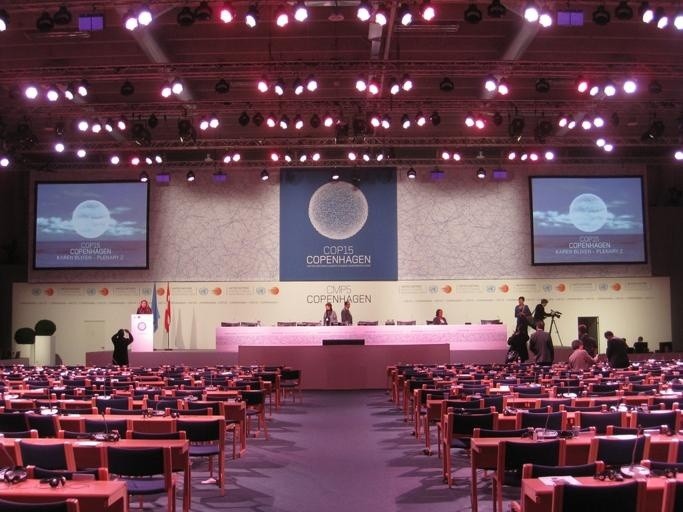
[441,410,683,481]
[426,394,682,454]
[0,477,128,512]
[597,352,682,365]
[519,471,682,512]
[0,381,272,420]
[0,414,225,496]
[0,389,262,438]
[215,324,507,352]
[413,383,682,442]
[469,431,682,512]
[0,399,247,458]
[0,436,189,512]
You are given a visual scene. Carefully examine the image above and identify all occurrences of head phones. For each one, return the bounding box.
[171,409,180,418]
[40,477,66,487]
[4,468,28,484]
[594,464,615,481]
[103,429,120,442]
[653,468,677,479]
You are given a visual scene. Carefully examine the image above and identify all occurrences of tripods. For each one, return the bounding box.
[549,317,562,346]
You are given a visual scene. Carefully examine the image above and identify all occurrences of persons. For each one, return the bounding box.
[111,328,134,366]
[534,299,554,319]
[137,300,152,314]
[604,331,628,368]
[341,301,352,324]
[568,340,598,369]
[515,296,532,334]
[578,324,598,358]
[323,302,337,326]
[530,320,554,366]
[507,324,529,364]
[433,309,448,325]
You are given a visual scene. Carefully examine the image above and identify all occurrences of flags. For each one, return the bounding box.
[165,282,172,331]
[151,284,160,333]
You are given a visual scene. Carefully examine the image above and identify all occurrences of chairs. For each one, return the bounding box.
[87,380,116,387]
[216,385,250,391]
[659,373,682,384]
[488,379,520,387]
[252,372,279,413]
[517,378,542,385]
[262,365,284,372]
[276,322,296,327]
[595,375,627,385]
[32,399,66,412]
[667,383,682,392]
[0,499,79,512]
[237,388,268,441]
[442,412,498,489]
[25,466,109,481]
[130,389,165,401]
[172,389,207,402]
[13,439,76,474]
[503,406,551,416]
[552,480,646,512]
[425,320,432,325]
[458,383,493,388]
[549,377,582,387]
[59,379,88,387]
[157,373,189,380]
[551,385,586,398]
[81,390,116,395]
[169,419,225,499]
[0,380,10,387]
[472,427,534,438]
[0,365,261,380]
[99,443,177,512]
[79,419,132,439]
[0,406,45,415]
[559,405,606,412]
[474,393,514,400]
[142,398,182,415]
[586,434,650,476]
[296,320,322,326]
[241,320,260,327]
[661,479,679,512]
[535,426,596,437]
[60,394,99,401]
[396,321,416,325]
[0,387,9,394]
[452,379,484,386]
[330,321,348,326]
[509,386,544,398]
[422,383,454,390]
[534,398,575,413]
[278,368,303,406]
[626,374,648,382]
[124,430,192,511]
[574,412,627,436]
[515,411,566,432]
[639,460,682,476]
[179,385,212,391]
[490,438,565,512]
[628,382,662,394]
[667,438,682,464]
[63,385,96,391]
[496,382,529,388]
[480,319,500,325]
[99,384,133,390]
[587,397,625,410]
[23,380,52,391]
[51,406,98,415]
[435,398,483,459]
[165,379,193,390]
[57,429,119,440]
[201,378,230,390]
[0,429,38,439]
[220,322,239,327]
[581,390,618,398]
[465,396,506,414]
[356,320,378,326]
[183,399,237,462]
[672,402,682,410]
[92,396,132,416]
[417,388,453,443]
[0,413,61,439]
[20,393,56,400]
[154,395,188,401]
[510,461,604,512]
[618,404,664,411]
[606,425,667,436]
[456,387,489,399]
[43,388,78,396]
[629,409,680,435]
[105,408,153,415]
[165,407,212,418]
[619,389,656,396]
[403,380,438,423]
[110,394,148,401]
[230,381,264,391]
[112,380,138,389]
[145,384,178,390]
[588,383,625,393]
[647,396,682,410]
[427,393,465,400]
[26,384,57,390]
[514,392,553,398]
[0,400,11,411]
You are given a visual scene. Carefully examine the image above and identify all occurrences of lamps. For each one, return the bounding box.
[104,117,114,132]
[160,79,172,98]
[218,2,234,23]
[362,150,373,163]
[280,115,289,130]
[256,73,270,92]
[138,3,152,26]
[464,112,475,127]
[273,1,288,27]
[475,148,485,159]
[399,114,410,129]
[373,150,383,162]
[407,167,416,179]
[646,120,665,141]
[25,82,38,99]
[283,150,295,163]
[370,113,381,127]
[322,113,332,127]
[76,79,88,96]
[348,149,358,160]
[441,150,451,161]
[356,0,372,21]
[45,85,60,101]
[272,77,285,95]
[522,0,539,22]
[572,73,587,92]
[601,76,615,97]
[396,1,413,26]
[671,6,682,30]
[452,149,464,161]
[329,169,339,179]
[77,116,88,131]
[579,115,592,130]
[198,116,208,130]
[64,82,75,100]
[482,72,498,92]
[260,169,268,180]
[229,151,241,162]
[381,114,391,129]
[139,170,148,182]
[350,168,361,182]
[308,150,320,161]
[0,10,10,31]
[355,71,366,91]
[295,150,307,163]
[267,114,278,128]
[90,117,101,133]
[144,153,156,165]
[415,113,425,127]
[367,76,381,94]
[565,113,575,130]
[245,0,260,28]
[554,113,567,128]
[539,0,554,28]
[117,116,127,131]
[120,7,138,30]
[497,76,510,95]
[290,77,304,95]
[155,153,166,163]
[304,73,317,91]
[186,170,194,182]
[218,151,230,164]
[398,73,412,91]
[374,0,391,26]
[652,5,668,30]
[516,148,528,161]
[585,81,599,96]
[208,114,219,129]
[592,112,604,127]
[502,148,514,159]
[475,168,486,179]
[622,74,637,93]
[270,150,282,161]
[418,0,435,21]
[387,76,398,95]
[291,115,304,130]
[125,153,140,166]
[475,113,489,131]
[170,76,183,95]
[637,0,653,24]
[292,0,308,22]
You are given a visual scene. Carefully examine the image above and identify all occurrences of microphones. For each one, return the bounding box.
[101,412,108,434]
[630,424,642,471]
[234,397,243,402]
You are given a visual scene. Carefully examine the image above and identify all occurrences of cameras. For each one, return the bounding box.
[550,309,562,319]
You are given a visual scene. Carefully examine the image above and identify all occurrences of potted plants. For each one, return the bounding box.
[34,319,56,366]
[14,327,35,366]
